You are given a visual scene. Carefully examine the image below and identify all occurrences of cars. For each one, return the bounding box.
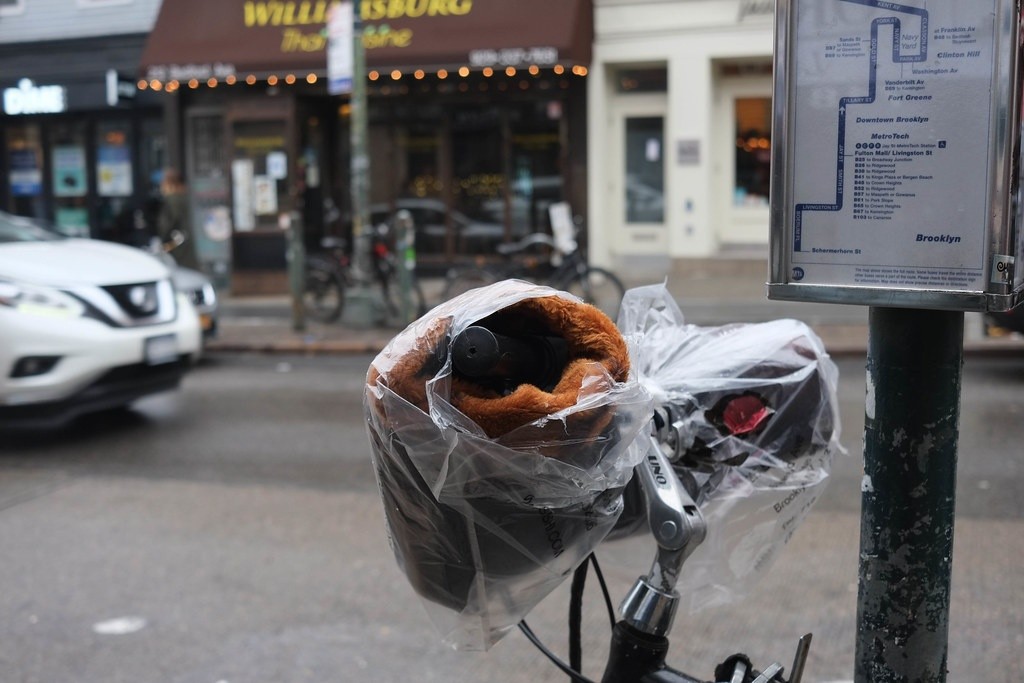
[325,197,514,256]
[0,208,206,434]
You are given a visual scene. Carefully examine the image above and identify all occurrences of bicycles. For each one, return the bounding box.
[439,232,628,327]
[297,228,427,331]
